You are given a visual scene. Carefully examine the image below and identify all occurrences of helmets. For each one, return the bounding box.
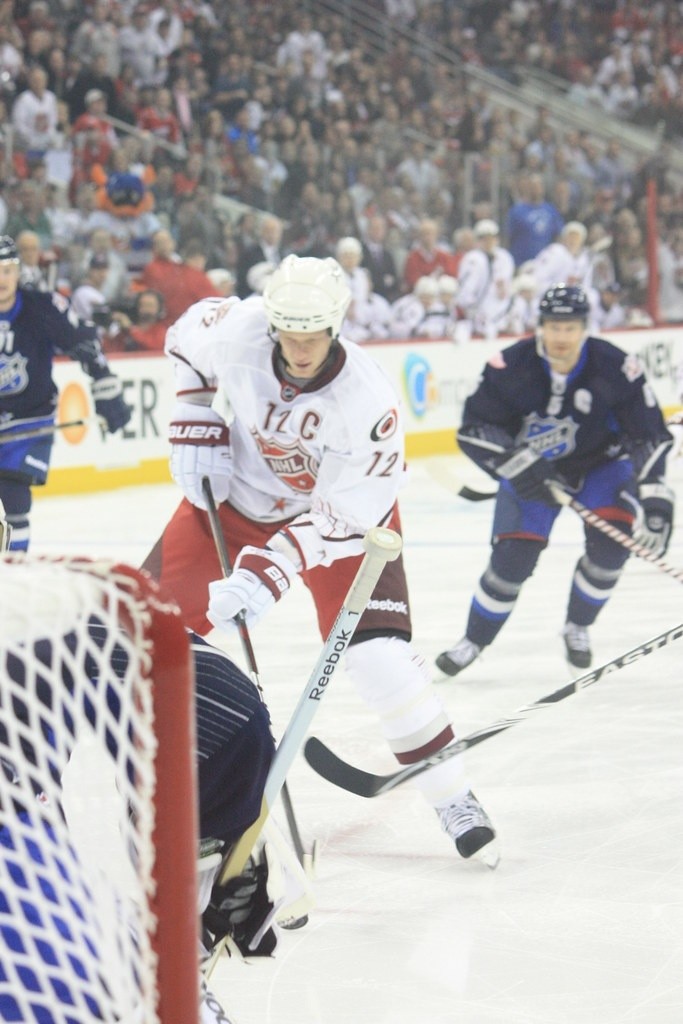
[538,285,590,320]
[261,253,353,339]
[0,236,20,265]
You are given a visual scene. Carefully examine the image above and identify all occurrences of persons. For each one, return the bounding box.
[0,236,131,556]
[137,254,501,868]
[435,285,677,676]
[0,618,277,962]
[1,1,683,349]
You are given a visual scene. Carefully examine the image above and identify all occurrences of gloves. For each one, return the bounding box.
[208,544,297,627]
[90,375,131,434]
[204,842,257,941]
[493,443,570,508]
[631,479,674,560]
[168,406,232,511]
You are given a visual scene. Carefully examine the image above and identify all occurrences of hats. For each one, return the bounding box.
[562,221,588,237]
[336,236,362,255]
[413,274,461,296]
[83,88,107,105]
[207,268,236,284]
[245,260,274,291]
[472,218,499,237]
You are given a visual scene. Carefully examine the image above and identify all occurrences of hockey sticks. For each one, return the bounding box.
[299,622,683,802]
[1,405,138,450]
[548,484,683,584]
[201,524,408,982]
[200,475,322,881]
[455,486,496,502]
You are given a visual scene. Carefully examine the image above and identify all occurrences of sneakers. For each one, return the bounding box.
[433,635,482,676]
[563,621,592,669]
[434,792,496,860]
